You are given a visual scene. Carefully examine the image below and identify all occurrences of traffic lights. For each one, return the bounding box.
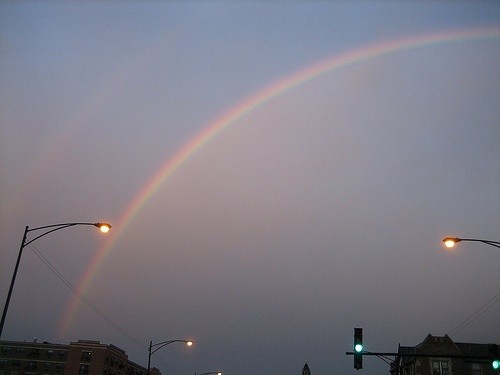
[354,327,364,368]
[1,220,113,334]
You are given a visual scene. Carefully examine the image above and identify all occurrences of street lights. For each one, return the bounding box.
[147,338,193,375]
